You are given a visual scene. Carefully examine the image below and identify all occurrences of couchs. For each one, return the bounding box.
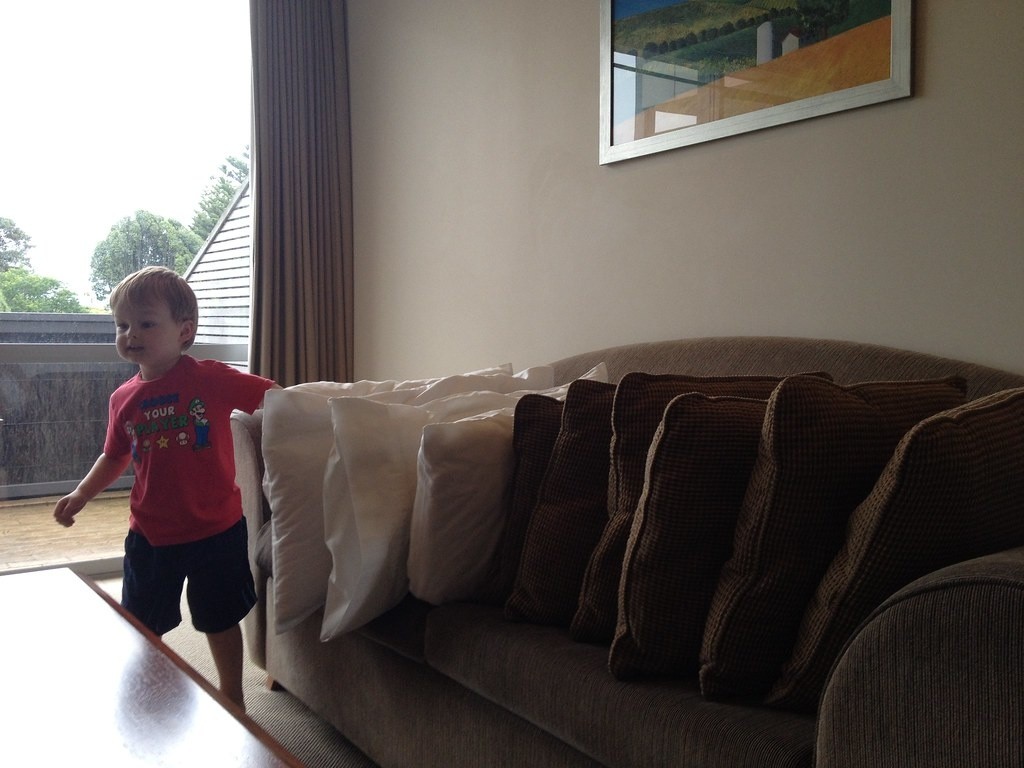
[228,336,1024,768]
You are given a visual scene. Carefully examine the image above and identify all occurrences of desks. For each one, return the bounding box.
[0,567,308,768]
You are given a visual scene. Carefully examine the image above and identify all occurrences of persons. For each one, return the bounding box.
[50,265,286,716]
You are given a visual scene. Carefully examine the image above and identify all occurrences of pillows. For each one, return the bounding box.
[257,355,1024,714]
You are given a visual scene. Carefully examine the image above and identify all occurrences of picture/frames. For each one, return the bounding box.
[599,0,911,164]
